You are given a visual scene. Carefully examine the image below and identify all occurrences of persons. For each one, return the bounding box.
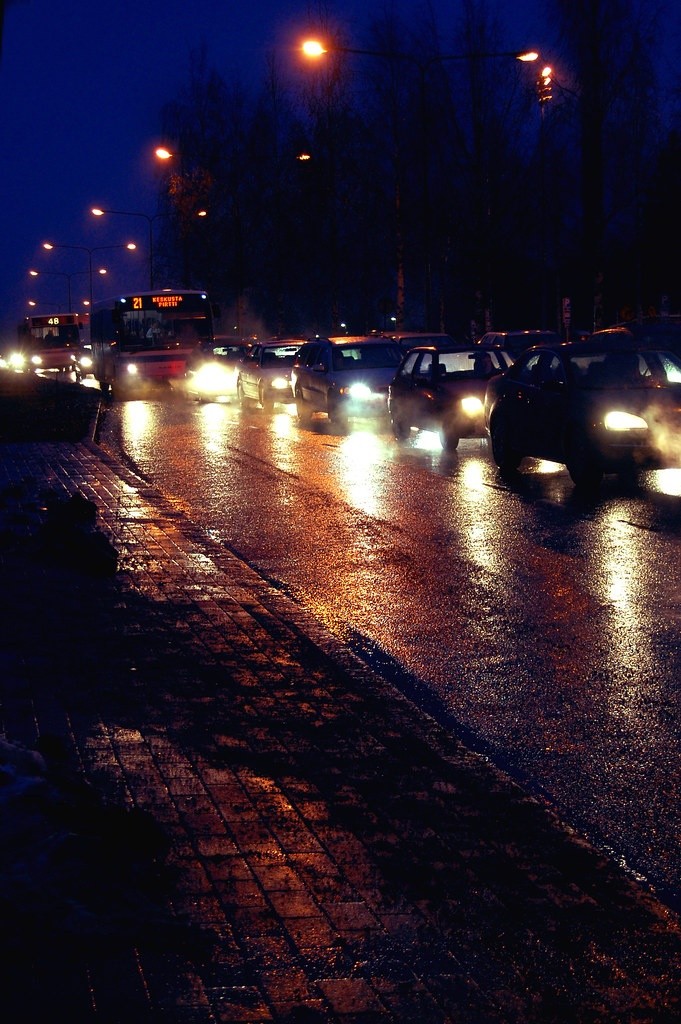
[123,314,199,338]
[45,330,75,349]
[607,360,667,390]
[474,353,500,381]
[464,319,480,344]
[586,288,610,335]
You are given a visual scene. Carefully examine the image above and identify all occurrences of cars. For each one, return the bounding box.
[479,330,569,366]
[288,331,413,421]
[372,330,454,360]
[479,342,680,491]
[384,343,516,453]
[211,330,310,368]
[586,317,681,363]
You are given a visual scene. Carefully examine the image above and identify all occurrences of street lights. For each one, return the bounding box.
[92,208,207,293]
[29,268,106,315]
[154,145,312,336]
[42,241,138,308]
[28,301,90,315]
[287,36,543,328]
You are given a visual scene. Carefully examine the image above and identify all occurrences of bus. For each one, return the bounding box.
[16,313,85,375]
[88,290,220,394]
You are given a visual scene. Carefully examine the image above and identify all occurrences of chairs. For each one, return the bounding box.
[590,362,609,389]
[262,352,278,369]
[427,363,448,382]
[552,362,584,386]
[530,364,553,387]
